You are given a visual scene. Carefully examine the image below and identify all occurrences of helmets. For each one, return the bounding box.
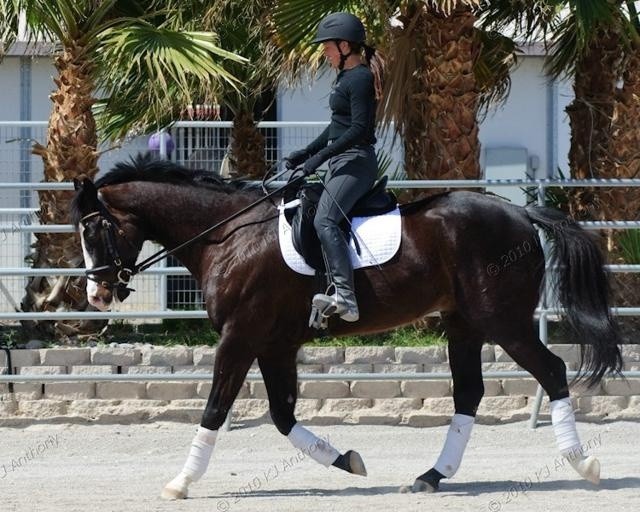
[307,12,367,45]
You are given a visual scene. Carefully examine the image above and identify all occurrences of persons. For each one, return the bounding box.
[284,12,383,323]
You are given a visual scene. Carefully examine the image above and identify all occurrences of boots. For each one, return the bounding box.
[312,224,360,322]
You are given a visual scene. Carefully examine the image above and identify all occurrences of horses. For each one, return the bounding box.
[66,149,632,502]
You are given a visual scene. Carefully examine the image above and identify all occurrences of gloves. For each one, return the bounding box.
[286,150,310,170]
[301,148,332,176]
